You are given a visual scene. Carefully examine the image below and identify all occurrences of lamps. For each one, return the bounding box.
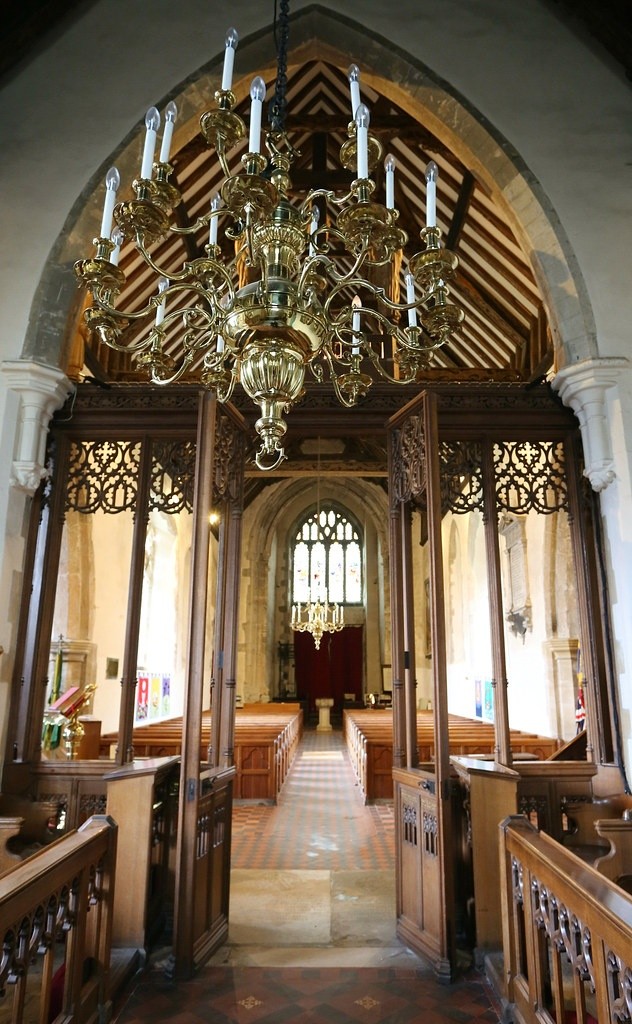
[70,0,467,472]
[283,580,349,650]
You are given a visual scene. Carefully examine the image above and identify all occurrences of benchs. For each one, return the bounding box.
[103,699,304,807]
[341,701,564,805]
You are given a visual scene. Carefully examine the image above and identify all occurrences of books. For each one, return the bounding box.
[49,688,81,711]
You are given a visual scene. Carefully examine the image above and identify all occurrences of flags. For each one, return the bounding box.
[575,641,586,734]
[42,647,62,750]
